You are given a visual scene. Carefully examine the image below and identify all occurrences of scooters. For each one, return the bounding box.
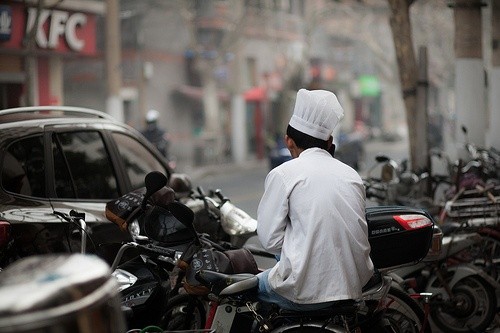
[0,125,500,333]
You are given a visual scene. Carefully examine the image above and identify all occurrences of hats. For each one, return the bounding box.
[289,89,345,141]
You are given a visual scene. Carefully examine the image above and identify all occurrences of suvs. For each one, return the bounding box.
[0,104,221,275]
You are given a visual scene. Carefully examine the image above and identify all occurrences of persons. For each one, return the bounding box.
[254,89,375,310]
[139,110,168,158]
